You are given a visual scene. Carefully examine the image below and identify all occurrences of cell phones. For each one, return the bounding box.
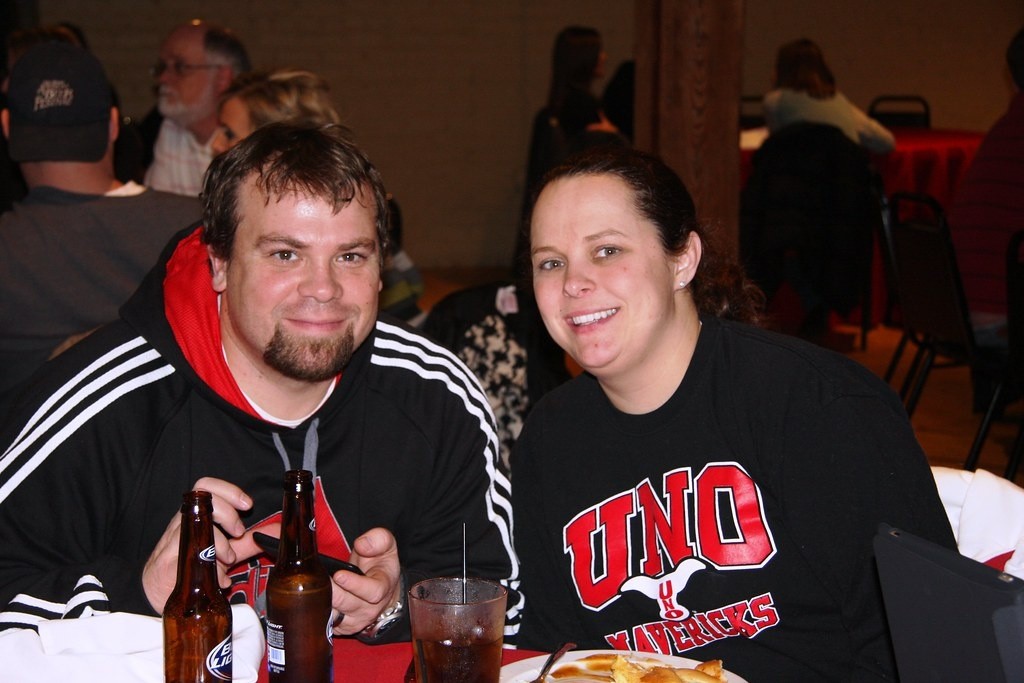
[252,531,365,582]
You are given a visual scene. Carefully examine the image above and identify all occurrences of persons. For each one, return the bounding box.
[511,143,961,683]
[211,66,341,156]
[0,116,525,651]
[523,26,634,143]
[0,21,206,455]
[757,40,897,330]
[941,27,1024,415]
[112,21,255,186]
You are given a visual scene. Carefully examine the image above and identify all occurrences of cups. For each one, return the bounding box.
[408,575,508,683]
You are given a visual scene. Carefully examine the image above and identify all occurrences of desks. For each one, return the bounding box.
[861,126,988,352]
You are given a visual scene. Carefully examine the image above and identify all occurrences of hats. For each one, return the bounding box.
[7,43,111,163]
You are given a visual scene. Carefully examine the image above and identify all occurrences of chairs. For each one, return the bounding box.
[966,232,1023,481]
[880,190,1009,419]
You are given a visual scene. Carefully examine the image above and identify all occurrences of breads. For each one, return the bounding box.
[609,655,731,683]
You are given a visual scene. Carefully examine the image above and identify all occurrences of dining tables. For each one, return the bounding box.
[254,630,550,683]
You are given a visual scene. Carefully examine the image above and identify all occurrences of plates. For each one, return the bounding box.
[500,649,749,683]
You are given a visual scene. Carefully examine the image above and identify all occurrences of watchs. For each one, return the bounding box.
[363,575,405,638]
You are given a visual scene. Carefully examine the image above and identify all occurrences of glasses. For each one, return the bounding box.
[153,60,225,77]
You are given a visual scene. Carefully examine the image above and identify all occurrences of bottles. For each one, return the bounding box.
[162,490,233,683]
[265,469,334,683]
